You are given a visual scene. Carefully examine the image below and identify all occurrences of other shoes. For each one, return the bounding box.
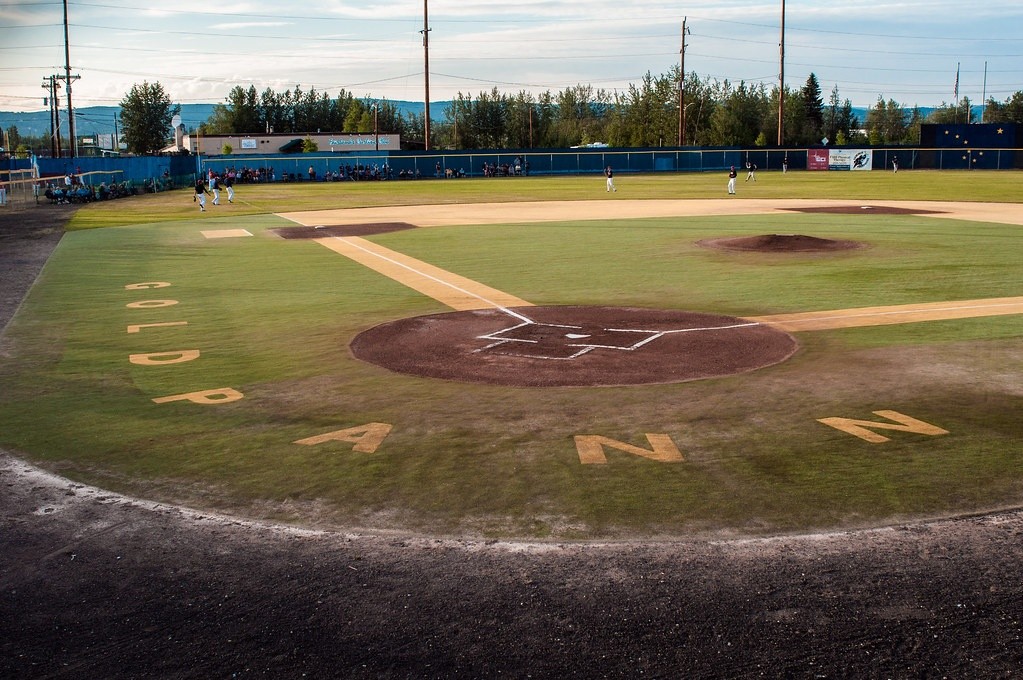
[212,202,215,205]
[200,204,203,208]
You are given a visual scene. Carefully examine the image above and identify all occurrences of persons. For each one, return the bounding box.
[482,161,521,178]
[445,168,465,178]
[746,162,757,181]
[147,169,170,193]
[224,174,233,203]
[892,155,899,173]
[339,164,357,181]
[358,163,380,181]
[194,180,211,211]
[212,178,222,205]
[32,176,41,197]
[208,166,274,192]
[326,171,338,181]
[782,157,789,173]
[400,169,422,179]
[282,171,288,183]
[45,167,137,205]
[436,162,440,178]
[727,166,737,194]
[309,165,316,181]
[382,162,394,180]
[604,166,617,192]
[0,178,7,203]
